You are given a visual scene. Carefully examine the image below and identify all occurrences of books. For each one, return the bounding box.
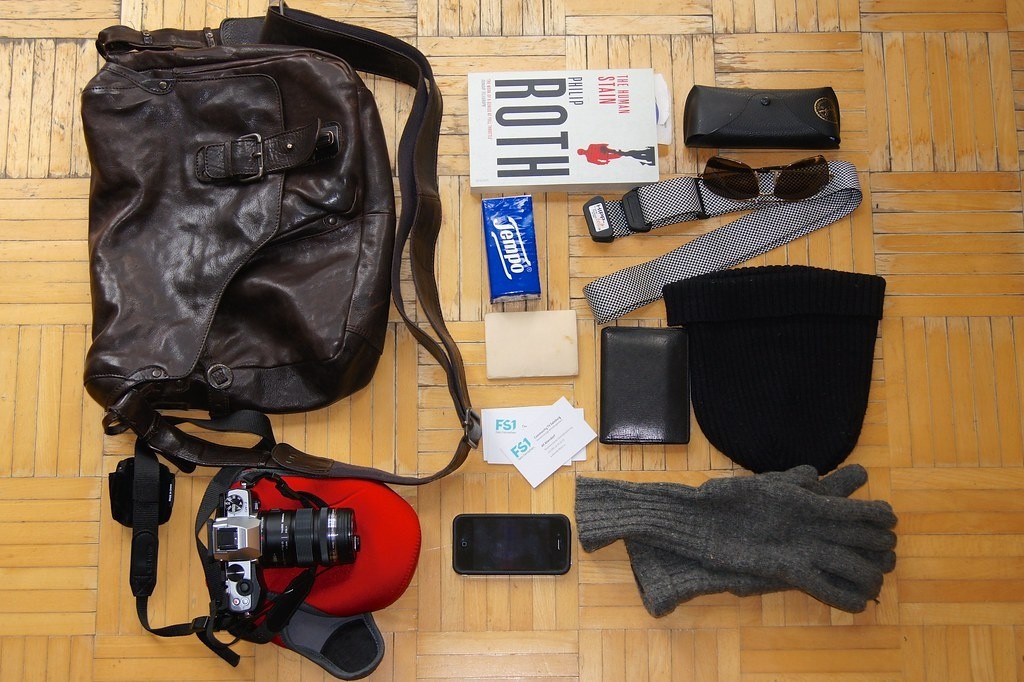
[468,67,659,193]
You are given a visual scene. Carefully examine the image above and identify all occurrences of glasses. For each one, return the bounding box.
[702,154,835,200]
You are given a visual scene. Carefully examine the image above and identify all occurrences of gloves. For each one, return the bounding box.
[573,463,898,619]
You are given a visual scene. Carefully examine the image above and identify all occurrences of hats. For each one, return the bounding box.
[661,264,886,476]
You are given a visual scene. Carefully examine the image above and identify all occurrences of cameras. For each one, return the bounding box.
[207,486,361,620]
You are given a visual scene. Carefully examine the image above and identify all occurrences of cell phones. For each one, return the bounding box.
[452,513,572,575]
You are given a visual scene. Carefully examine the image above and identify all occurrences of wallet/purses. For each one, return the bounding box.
[599,326,691,445]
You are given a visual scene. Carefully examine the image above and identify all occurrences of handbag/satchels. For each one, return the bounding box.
[81,43,396,419]
